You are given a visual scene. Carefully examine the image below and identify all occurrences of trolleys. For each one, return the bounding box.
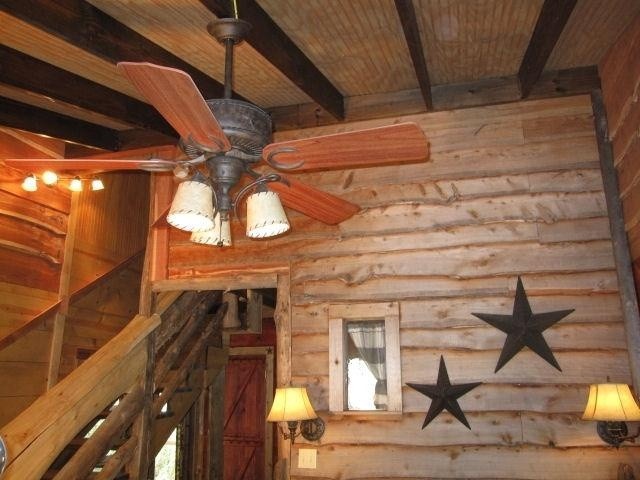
[5,0,430,226]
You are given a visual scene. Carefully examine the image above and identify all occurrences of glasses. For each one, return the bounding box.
[166,155,291,248]
[581,383,640,445]
[266,388,325,444]
[21,170,105,191]
